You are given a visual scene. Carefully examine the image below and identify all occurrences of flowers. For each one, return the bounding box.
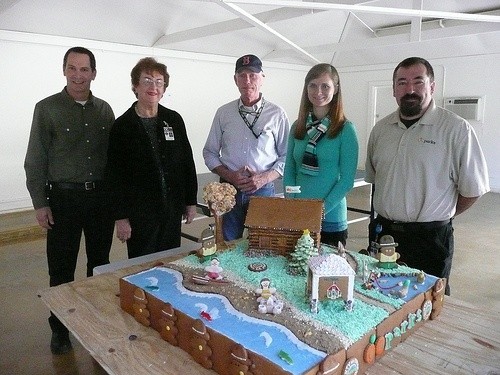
[202,182,237,216]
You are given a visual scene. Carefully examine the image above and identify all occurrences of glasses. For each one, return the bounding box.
[138,79,166,88]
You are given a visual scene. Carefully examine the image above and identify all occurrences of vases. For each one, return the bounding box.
[214,215,224,243]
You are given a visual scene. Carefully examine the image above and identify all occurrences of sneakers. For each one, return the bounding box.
[51,331,71,354]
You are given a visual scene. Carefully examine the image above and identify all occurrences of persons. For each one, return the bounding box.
[24,47,116,353]
[285,63,359,249]
[203,54,289,240]
[363,57,491,297]
[108,58,198,259]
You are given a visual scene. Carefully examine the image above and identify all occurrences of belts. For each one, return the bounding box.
[377,215,451,232]
[52,182,104,191]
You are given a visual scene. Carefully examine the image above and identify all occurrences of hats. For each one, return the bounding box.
[235,54,262,73]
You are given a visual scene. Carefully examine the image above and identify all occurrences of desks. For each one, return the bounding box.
[197,170,372,225]
[36,243,500,375]
